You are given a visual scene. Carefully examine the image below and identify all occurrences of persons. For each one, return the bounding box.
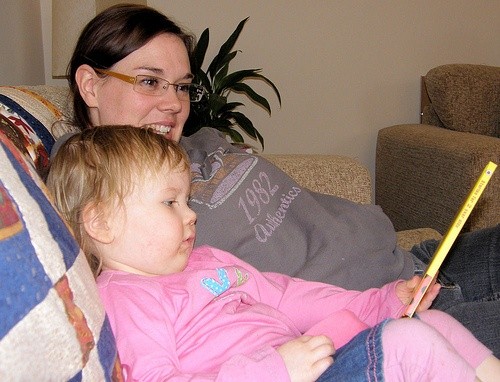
[46,124,500,382]
[65,3,499,360]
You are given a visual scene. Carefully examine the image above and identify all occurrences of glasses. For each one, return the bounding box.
[94,67,204,102]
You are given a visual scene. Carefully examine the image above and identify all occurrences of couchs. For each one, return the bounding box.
[375,63,500,235]
[0,84,444,381]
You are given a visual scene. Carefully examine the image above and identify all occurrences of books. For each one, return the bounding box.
[402,161,498,318]
